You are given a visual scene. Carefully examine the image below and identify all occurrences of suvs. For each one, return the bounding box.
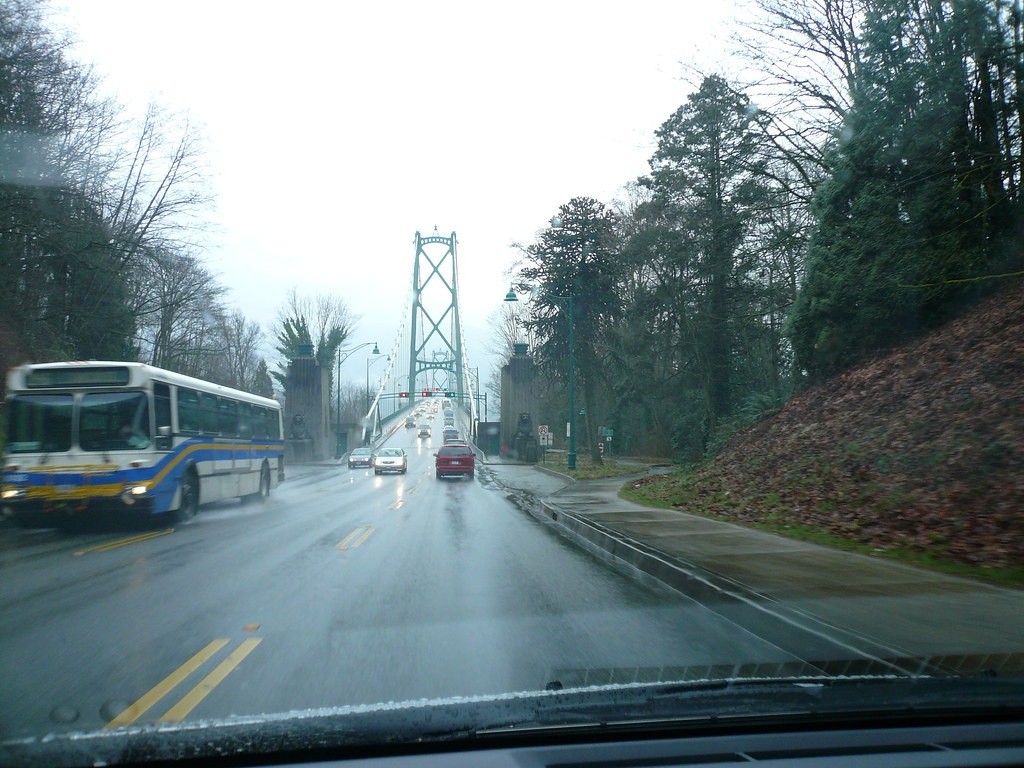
[443,429,459,442]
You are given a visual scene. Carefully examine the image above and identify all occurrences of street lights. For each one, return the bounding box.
[449,361,479,419]
[418,381,423,400]
[367,354,391,415]
[334,342,380,460]
[415,379,418,381]
[504,282,576,470]
[394,375,410,413]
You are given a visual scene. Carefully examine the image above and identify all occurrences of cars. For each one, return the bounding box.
[348,448,377,468]
[444,439,465,444]
[404,399,455,428]
[374,448,407,475]
[433,444,476,481]
[417,424,431,437]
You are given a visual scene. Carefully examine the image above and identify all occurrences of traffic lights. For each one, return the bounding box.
[445,392,455,397]
[444,388,449,391]
[433,388,438,391]
[422,392,432,397]
[424,388,429,391]
[399,392,409,397]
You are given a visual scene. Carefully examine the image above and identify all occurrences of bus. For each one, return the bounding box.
[0,360,285,525]
